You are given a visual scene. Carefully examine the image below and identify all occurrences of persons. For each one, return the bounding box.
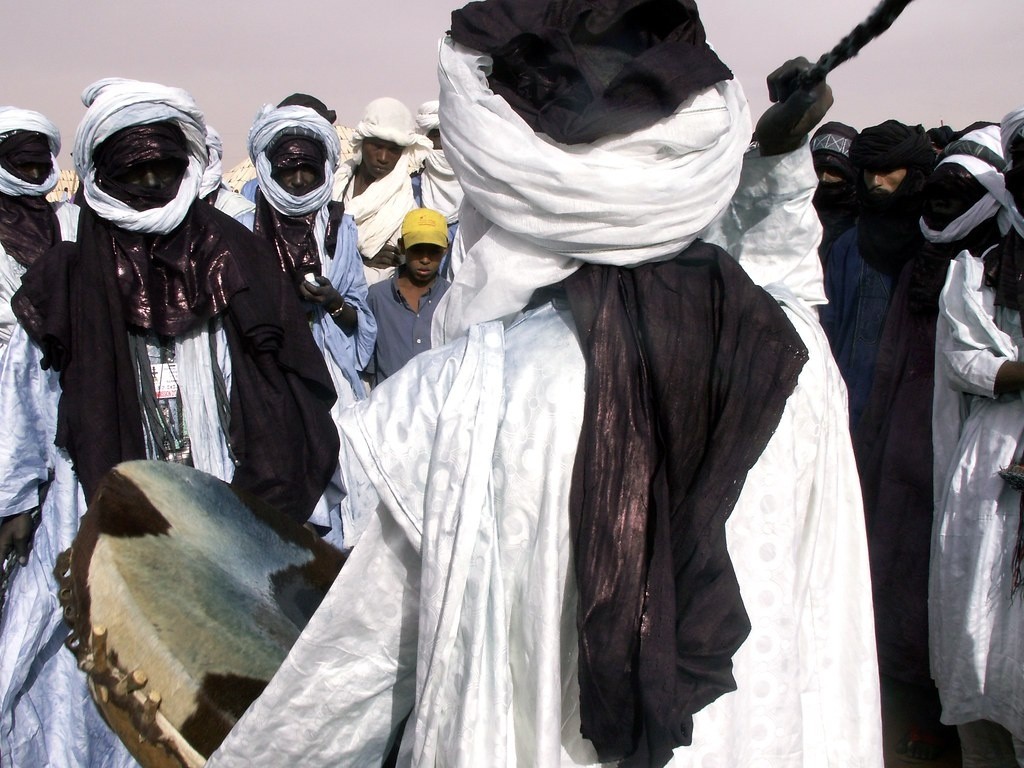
[0,76,341,768]
[199,0,884,768]
[201,94,1024,768]
[0,107,80,344]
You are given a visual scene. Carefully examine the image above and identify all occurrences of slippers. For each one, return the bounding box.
[896,706,952,764]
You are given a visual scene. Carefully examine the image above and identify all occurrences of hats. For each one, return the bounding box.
[401,208,449,249]
